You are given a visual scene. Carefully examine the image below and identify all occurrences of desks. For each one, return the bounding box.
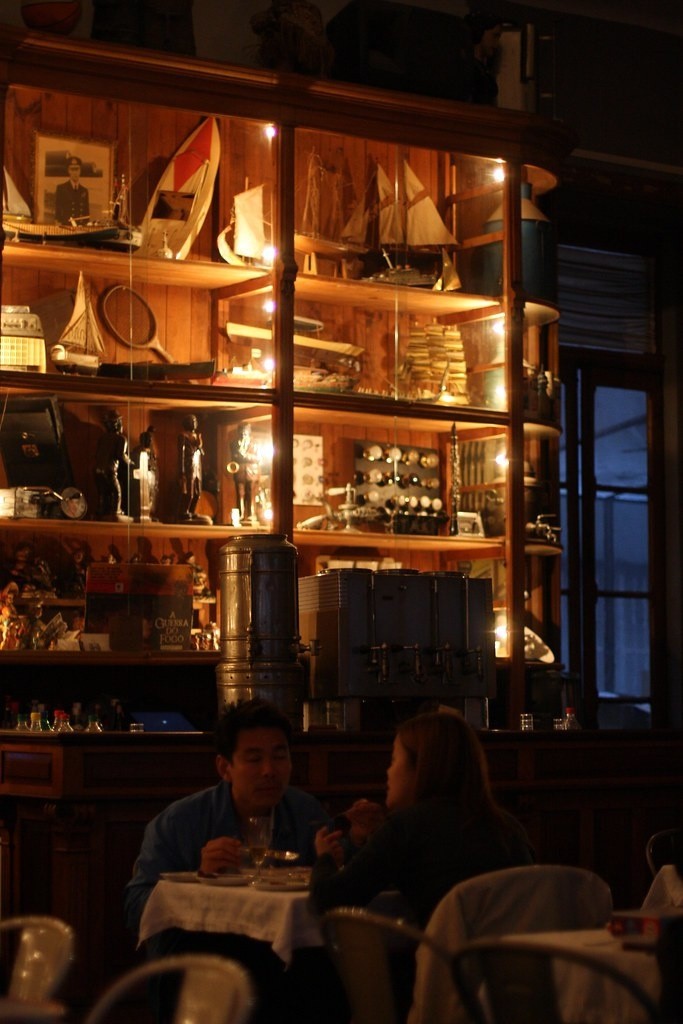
[481,928,662,1024]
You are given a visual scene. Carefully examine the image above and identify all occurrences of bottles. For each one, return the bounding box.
[0,696,125,731]
[563,708,579,730]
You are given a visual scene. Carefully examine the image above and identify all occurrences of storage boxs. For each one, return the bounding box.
[80,559,193,656]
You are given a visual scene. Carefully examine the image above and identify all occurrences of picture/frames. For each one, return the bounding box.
[27,122,119,228]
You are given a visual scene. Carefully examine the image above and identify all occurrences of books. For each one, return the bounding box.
[484,241,658,355]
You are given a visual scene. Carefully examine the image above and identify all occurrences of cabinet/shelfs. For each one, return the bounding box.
[0,20,570,675]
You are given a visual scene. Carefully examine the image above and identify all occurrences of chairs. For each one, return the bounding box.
[88,952,255,1024]
[0,913,74,1024]
[317,906,484,1024]
[406,862,615,1024]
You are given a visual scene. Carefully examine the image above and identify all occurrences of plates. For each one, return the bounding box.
[255,882,310,890]
[161,872,198,882]
[199,878,250,886]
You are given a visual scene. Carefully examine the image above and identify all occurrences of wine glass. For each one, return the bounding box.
[241,816,273,886]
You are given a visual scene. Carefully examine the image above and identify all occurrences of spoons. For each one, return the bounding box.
[239,849,299,861]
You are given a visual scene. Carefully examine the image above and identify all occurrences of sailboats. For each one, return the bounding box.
[379,318,471,405]
[217,144,462,292]
[51,270,108,376]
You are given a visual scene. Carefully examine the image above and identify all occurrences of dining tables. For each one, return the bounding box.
[136,866,326,971]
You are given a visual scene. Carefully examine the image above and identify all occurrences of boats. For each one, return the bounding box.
[127,116,221,260]
[2,167,142,254]
[211,313,366,393]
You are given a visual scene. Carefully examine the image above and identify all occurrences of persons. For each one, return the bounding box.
[93,409,134,516]
[131,429,159,514]
[54,157,91,227]
[178,414,206,520]
[444,10,505,105]
[307,709,538,1023]
[228,421,256,519]
[120,694,382,1024]
[8,544,207,592]
[0,582,25,639]
